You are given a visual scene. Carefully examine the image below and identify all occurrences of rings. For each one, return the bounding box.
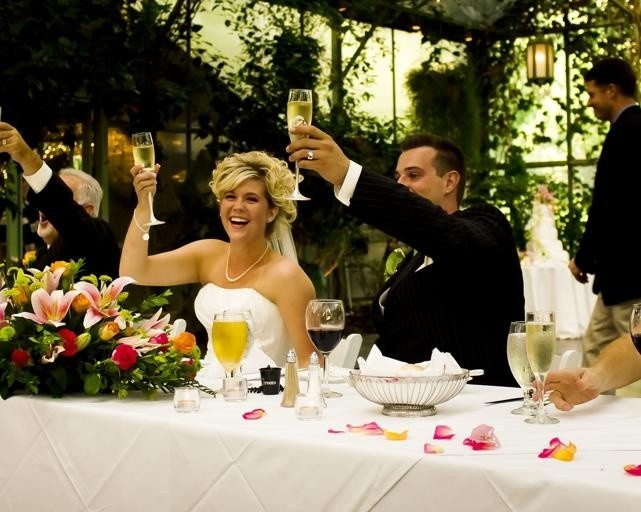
[308,152,313,161]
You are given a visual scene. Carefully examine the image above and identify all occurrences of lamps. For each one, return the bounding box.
[526,29,554,87]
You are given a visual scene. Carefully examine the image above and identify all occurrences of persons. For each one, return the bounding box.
[532,321,641,412]
[119,149,324,394]
[286,125,525,388]
[0,122,119,288]
[567,56,641,396]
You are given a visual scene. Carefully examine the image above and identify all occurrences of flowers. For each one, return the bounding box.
[0,250,203,401]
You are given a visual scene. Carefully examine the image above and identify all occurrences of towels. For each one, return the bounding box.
[357,344,462,376]
[280,333,363,383]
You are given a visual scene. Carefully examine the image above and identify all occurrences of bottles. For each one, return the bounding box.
[280,349,299,407]
[304,353,327,411]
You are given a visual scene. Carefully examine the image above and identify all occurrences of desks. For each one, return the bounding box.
[520,260,600,340]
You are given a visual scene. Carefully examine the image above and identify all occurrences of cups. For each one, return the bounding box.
[296,391,323,422]
[220,378,248,403]
[259,366,281,396]
[171,387,201,413]
[629,304,641,359]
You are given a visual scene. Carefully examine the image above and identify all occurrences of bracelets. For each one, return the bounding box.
[133,209,151,241]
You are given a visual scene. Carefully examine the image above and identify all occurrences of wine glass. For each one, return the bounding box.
[131,132,166,227]
[211,313,248,379]
[506,321,540,416]
[525,311,559,425]
[225,308,255,388]
[282,87,313,199]
[304,299,345,399]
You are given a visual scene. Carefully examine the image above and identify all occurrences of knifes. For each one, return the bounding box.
[484,397,523,405]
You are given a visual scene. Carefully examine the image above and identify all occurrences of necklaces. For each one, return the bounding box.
[226,243,269,282]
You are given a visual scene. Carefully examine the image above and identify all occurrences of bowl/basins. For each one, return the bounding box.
[346,369,468,419]
